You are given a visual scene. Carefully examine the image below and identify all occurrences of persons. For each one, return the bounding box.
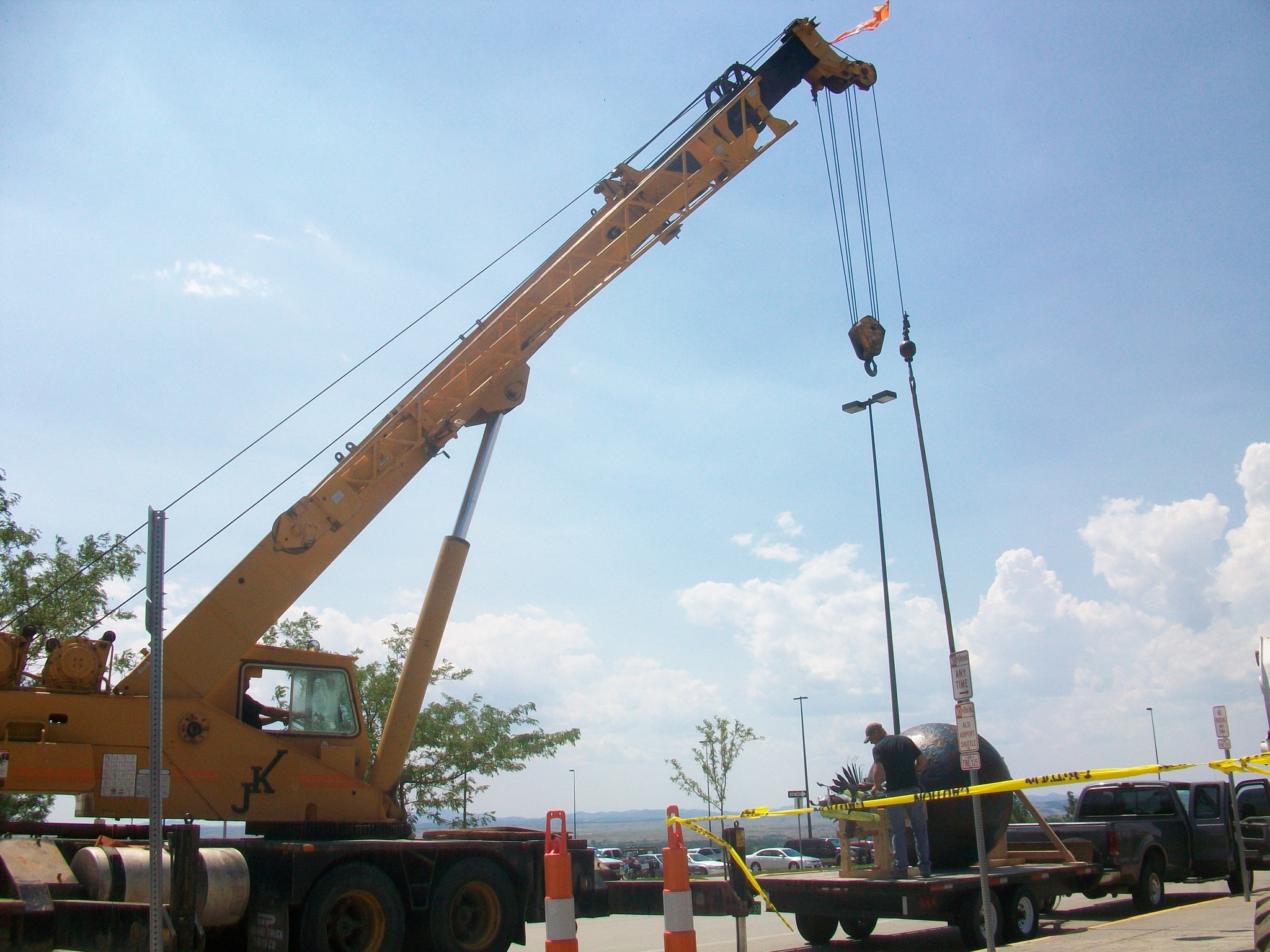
[241,671,308,731]
[864,723,932,878]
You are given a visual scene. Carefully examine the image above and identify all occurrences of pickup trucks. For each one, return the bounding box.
[1008,778,1269,912]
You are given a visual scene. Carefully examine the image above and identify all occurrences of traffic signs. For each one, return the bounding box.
[949,649,974,701]
[788,790,808,798]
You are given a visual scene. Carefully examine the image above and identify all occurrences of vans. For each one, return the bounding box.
[598,848,622,860]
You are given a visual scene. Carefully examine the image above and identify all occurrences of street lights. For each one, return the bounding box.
[569,769,576,839]
[792,696,813,839]
[1147,707,1160,779]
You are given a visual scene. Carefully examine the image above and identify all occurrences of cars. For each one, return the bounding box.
[849,840,894,860]
[627,854,664,879]
[745,848,822,872]
[686,853,731,877]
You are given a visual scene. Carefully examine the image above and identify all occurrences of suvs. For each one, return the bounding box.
[621,846,658,870]
[783,838,867,866]
[687,847,724,862]
[587,847,625,881]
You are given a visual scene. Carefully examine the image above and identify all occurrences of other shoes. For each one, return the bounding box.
[920,868,930,878]
[891,870,909,879]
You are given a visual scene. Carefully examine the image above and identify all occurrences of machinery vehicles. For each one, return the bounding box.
[1,15,882,952]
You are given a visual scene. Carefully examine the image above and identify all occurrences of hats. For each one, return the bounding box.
[865,723,881,744]
